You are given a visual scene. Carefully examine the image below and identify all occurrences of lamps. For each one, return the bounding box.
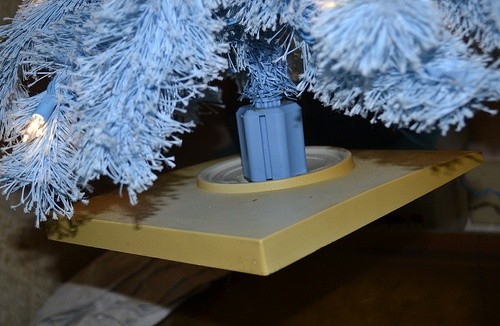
[19,71,69,144]
